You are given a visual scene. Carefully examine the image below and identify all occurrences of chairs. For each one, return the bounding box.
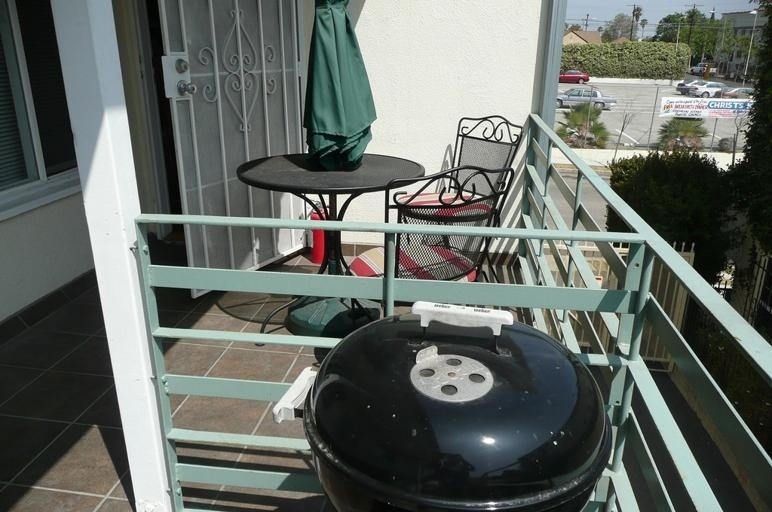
[384,114,521,283]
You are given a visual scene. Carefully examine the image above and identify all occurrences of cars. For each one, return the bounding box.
[558,68,592,86]
[675,61,756,100]
[555,86,618,113]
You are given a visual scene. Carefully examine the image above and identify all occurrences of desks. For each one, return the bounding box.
[237,152,425,346]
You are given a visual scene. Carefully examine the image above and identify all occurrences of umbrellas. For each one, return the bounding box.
[284,0,382,336]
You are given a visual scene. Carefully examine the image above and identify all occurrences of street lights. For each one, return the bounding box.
[708,11,726,48]
[741,10,759,87]
[677,15,688,45]
